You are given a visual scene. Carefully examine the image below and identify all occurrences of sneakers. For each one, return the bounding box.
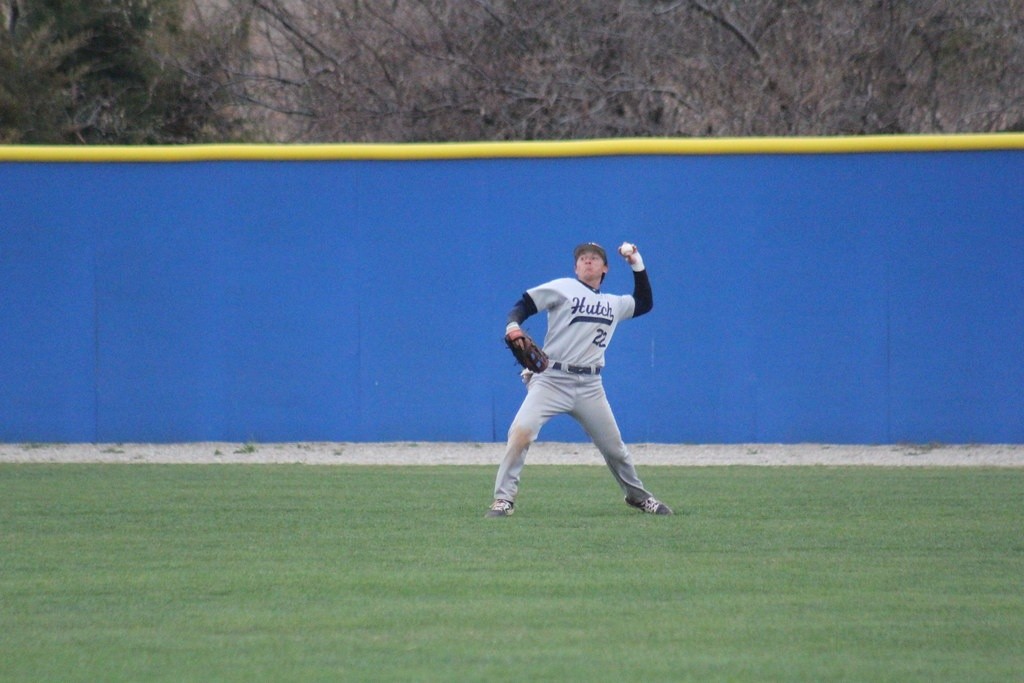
[486,499,514,518]
[624,495,673,515]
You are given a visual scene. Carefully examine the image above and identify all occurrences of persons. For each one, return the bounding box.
[483,240,674,517]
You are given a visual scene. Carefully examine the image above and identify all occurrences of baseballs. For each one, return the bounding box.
[621,243,633,256]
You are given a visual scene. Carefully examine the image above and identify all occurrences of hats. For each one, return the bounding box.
[574,241,607,265]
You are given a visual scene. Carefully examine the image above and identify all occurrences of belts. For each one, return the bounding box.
[553,362,600,374]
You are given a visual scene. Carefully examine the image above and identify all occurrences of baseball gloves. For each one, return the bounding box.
[504,327,550,374]
